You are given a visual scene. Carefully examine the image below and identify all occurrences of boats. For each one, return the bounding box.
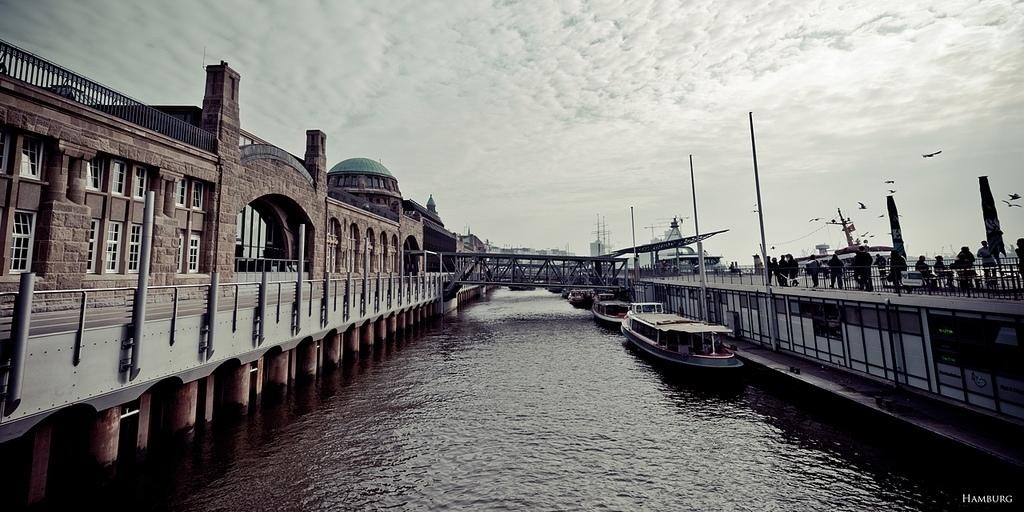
[567,289,596,308]
[620,302,746,369]
[592,293,628,330]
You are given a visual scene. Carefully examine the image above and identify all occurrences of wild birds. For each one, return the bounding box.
[831,219,836,224]
[860,231,875,238]
[778,272,803,287]
[808,218,824,222]
[885,180,895,183]
[857,201,867,210]
[920,150,942,158]
[1001,192,1023,207]
[888,189,896,194]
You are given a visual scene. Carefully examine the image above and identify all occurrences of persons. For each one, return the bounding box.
[767,238,1024,291]
[729,261,738,273]
